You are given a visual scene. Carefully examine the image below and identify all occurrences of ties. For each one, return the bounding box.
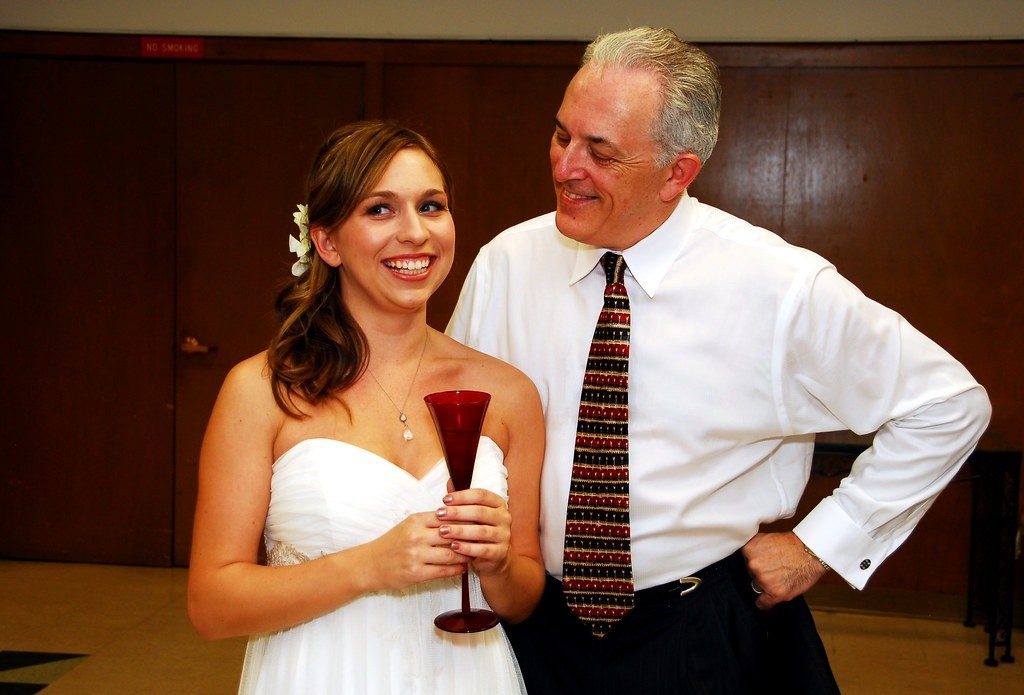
[561,253,636,639]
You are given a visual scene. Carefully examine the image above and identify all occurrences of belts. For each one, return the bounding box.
[545,548,743,610]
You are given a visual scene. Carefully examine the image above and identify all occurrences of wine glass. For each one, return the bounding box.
[423,390,501,635]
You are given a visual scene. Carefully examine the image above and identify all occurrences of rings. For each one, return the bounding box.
[751,582,762,594]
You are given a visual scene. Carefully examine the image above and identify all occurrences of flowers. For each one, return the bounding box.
[288,203,309,278]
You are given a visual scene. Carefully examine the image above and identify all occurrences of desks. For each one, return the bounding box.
[813,441,1023,666]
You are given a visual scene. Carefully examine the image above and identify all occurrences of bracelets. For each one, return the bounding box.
[804,547,830,571]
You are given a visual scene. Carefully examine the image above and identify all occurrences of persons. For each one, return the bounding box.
[444,27,992,695]
[188,120,547,695]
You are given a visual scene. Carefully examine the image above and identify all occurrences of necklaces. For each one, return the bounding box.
[362,323,428,442]
